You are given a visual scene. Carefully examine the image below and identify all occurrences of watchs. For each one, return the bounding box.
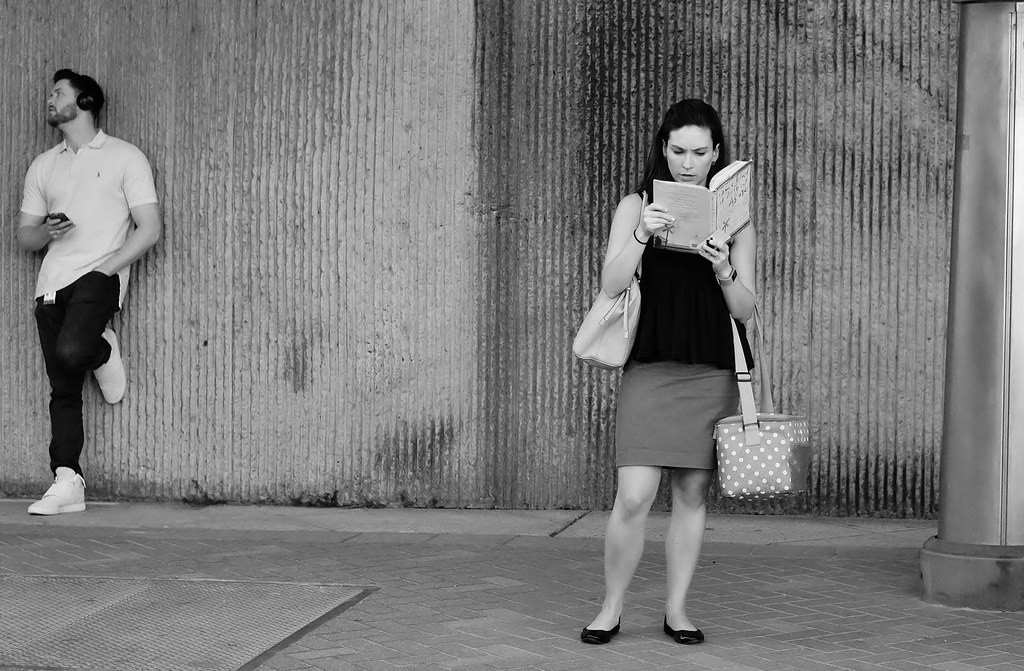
[715,265,738,286]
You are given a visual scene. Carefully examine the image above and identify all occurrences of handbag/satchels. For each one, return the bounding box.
[572,191,649,370]
[713,413,810,502]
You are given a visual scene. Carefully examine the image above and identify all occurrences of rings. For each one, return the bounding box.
[57,230,60,234]
[716,253,720,258]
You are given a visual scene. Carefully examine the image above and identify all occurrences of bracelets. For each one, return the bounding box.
[634,229,648,245]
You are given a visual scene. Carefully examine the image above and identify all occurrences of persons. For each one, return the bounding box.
[16,69,162,516]
[580,99,758,646]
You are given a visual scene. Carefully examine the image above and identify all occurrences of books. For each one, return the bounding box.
[653,160,751,254]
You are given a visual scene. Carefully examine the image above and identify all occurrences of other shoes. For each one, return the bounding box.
[581,616,620,644]
[664,614,704,644]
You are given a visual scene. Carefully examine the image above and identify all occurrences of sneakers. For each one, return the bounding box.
[27,466,85,515]
[93,329,126,404]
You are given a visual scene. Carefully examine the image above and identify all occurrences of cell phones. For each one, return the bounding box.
[48,212,72,231]
